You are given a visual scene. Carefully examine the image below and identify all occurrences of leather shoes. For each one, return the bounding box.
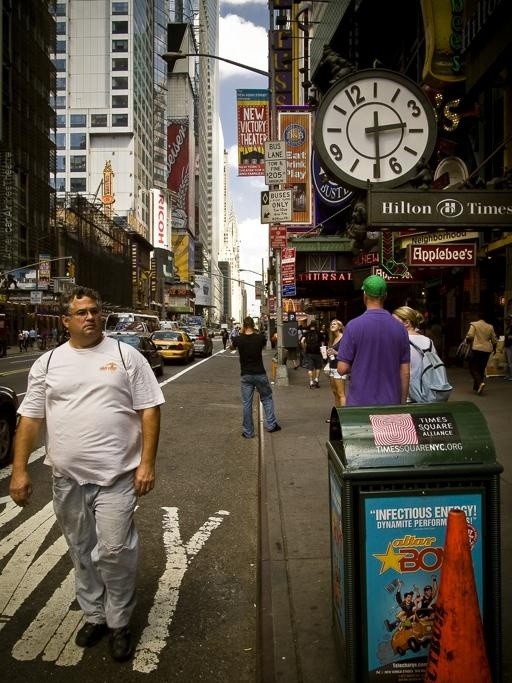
[76,621,106,645]
[107,621,134,661]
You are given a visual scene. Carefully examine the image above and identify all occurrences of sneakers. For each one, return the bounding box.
[314,379,321,389]
[268,423,281,432]
[476,381,485,394]
[307,384,314,389]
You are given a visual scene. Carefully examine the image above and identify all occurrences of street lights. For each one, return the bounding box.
[239,258,266,316]
[161,31,290,388]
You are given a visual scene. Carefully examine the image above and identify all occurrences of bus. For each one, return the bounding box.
[105,312,161,335]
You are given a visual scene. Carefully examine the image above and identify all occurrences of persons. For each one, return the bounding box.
[51,325,57,340]
[271,328,277,348]
[325,319,348,422]
[229,316,281,438]
[230,325,241,343]
[8,284,160,662]
[502,316,512,382]
[65,329,71,341]
[391,306,436,404]
[426,316,441,358]
[18,327,35,353]
[220,328,229,351]
[41,327,48,336]
[407,573,438,617]
[254,326,268,340]
[466,312,498,395]
[336,275,412,410]
[291,319,330,389]
[383,578,418,633]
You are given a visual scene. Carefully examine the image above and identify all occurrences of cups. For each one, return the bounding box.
[327,347,335,361]
[320,345,327,359]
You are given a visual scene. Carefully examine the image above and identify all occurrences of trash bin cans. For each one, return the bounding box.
[326,401,504,682]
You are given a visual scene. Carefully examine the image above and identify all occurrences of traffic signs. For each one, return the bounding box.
[260,189,292,221]
[30,291,43,304]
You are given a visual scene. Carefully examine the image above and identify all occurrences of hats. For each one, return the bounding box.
[361,274,389,297]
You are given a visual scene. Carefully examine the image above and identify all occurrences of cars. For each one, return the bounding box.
[149,328,197,362]
[104,334,166,379]
[0,386,23,468]
[161,319,182,331]
[205,325,234,336]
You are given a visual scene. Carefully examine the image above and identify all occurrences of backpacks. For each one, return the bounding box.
[407,334,452,403]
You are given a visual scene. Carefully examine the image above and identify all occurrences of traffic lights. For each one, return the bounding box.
[189,274,194,288]
[72,265,76,278]
[66,263,71,278]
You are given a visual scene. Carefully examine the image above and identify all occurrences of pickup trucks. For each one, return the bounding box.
[109,320,152,338]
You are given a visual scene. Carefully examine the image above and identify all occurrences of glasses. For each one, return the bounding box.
[65,309,103,320]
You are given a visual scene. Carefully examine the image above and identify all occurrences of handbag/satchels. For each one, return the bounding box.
[456,338,472,359]
[319,346,326,361]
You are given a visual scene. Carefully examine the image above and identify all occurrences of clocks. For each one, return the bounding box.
[310,64,441,193]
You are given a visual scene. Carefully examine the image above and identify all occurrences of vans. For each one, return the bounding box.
[177,322,214,358]
